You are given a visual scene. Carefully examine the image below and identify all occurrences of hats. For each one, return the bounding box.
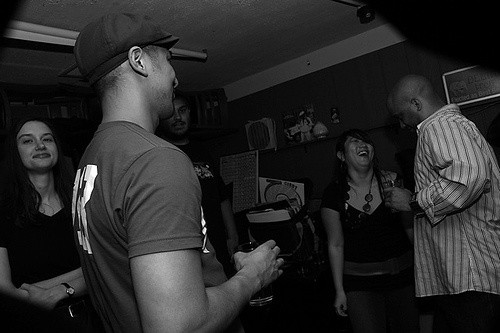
[74,11,180,84]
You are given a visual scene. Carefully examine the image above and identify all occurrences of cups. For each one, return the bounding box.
[234,241,274,307]
[383,179,404,212]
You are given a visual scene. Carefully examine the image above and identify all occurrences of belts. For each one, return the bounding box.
[344,250,417,277]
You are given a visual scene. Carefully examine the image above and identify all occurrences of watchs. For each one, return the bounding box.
[409,192,424,214]
[60,283,75,296]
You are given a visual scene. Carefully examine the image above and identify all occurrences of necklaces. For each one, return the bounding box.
[345,167,374,226]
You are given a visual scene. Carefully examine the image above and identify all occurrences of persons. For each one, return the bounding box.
[159,90,240,280]
[0,118,105,333]
[70,12,285,333]
[320,130,422,333]
[384,74,500,333]
